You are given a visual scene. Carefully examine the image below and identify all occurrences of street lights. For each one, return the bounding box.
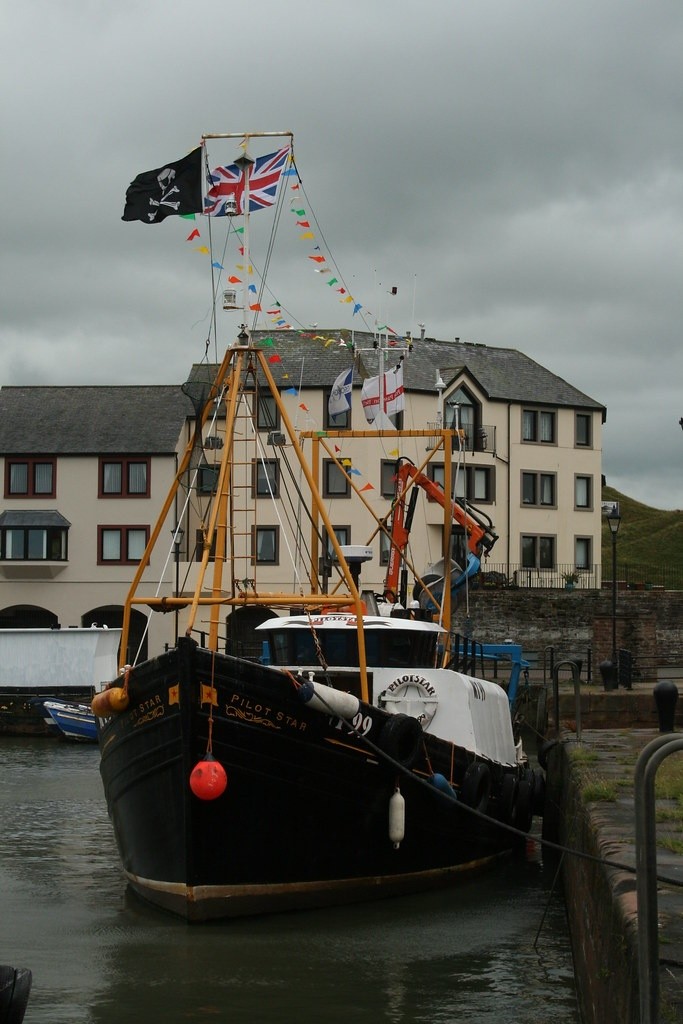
[169,521,185,647]
[608,505,623,687]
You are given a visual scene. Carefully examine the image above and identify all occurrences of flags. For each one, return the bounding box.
[120,145,203,225]
[329,368,353,416]
[362,359,405,424]
[200,145,291,216]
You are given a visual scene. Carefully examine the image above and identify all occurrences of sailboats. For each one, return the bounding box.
[91,132,543,927]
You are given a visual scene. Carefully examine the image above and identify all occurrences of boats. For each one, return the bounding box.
[26,697,97,744]
